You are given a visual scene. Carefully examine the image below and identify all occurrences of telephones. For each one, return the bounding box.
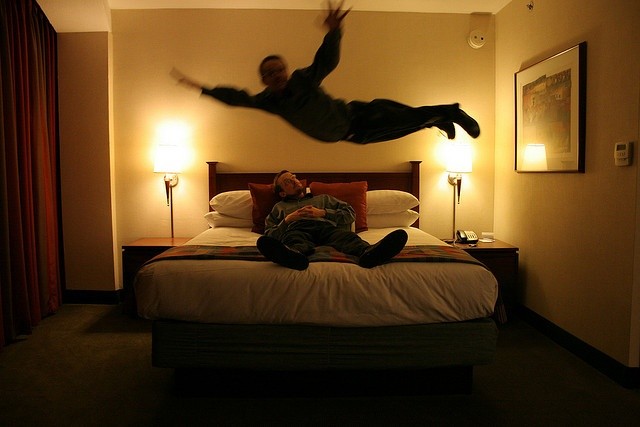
[456,230,478,244]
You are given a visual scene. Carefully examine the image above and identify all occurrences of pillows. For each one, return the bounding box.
[367,210,421,227]
[309,181,369,232]
[364,189,421,213]
[247,180,307,231]
[209,191,253,220]
[203,212,253,227]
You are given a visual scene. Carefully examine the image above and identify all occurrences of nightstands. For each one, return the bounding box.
[440,236,520,331]
[122,238,191,316]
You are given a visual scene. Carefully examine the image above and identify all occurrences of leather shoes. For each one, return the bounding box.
[257,236,309,270]
[454,110,479,138]
[439,122,455,139]
[357,229,407,269]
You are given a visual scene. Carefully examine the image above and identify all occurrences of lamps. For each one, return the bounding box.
[155,144,181,238]
[523,144,548,171]
[446,142,475,240]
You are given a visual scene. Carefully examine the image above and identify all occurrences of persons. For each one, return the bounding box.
[256,169,409,271]
[168,0,480,145]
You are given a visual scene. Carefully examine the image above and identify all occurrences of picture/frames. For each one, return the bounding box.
[514,42,586,174]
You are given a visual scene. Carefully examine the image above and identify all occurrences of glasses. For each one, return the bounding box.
[260,64,287,78]
[280,174,297,190]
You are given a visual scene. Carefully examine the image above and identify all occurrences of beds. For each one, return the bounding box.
[136,161,499,405]
